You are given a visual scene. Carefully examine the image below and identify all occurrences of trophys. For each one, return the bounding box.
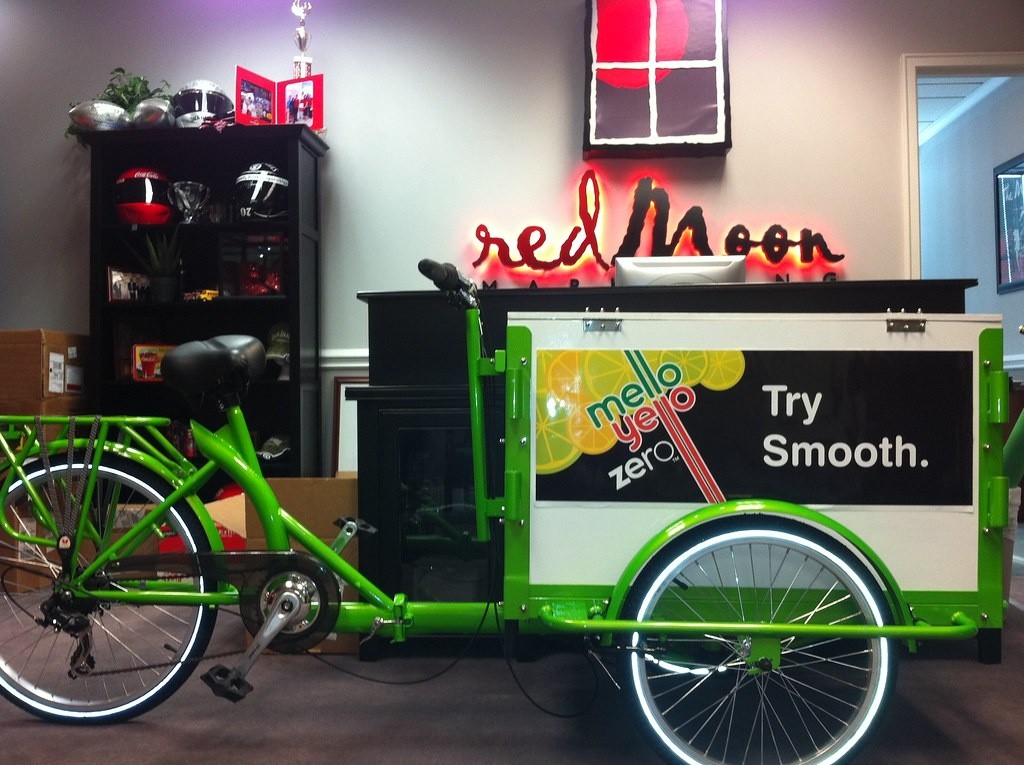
[291,0,326,135]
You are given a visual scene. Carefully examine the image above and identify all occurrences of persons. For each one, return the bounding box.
[241,83,271,122]
[287,91,313,123]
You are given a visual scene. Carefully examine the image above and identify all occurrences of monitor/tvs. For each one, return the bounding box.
[613,254,747,287]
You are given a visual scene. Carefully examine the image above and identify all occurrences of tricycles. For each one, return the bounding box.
[1,258,1012,765]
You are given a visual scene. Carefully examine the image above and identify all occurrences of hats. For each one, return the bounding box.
[255,433,290,458]
[264,324,290,361]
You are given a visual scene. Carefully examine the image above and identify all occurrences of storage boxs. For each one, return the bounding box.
[0,329,89,455]
[0,481,98,593]
[247,472,359,654]
[107,503,160,580]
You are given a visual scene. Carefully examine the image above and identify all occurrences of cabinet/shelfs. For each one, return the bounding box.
[67,125,330,533]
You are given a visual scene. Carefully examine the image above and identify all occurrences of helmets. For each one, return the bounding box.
[235,162,289,219]
[167,181,211,215]
[173,79,234,127]
[114,168,173,224]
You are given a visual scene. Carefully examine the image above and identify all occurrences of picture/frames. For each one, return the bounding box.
[992,152,1024,295]
[276,73,324,131]
[235,66,276,126]
